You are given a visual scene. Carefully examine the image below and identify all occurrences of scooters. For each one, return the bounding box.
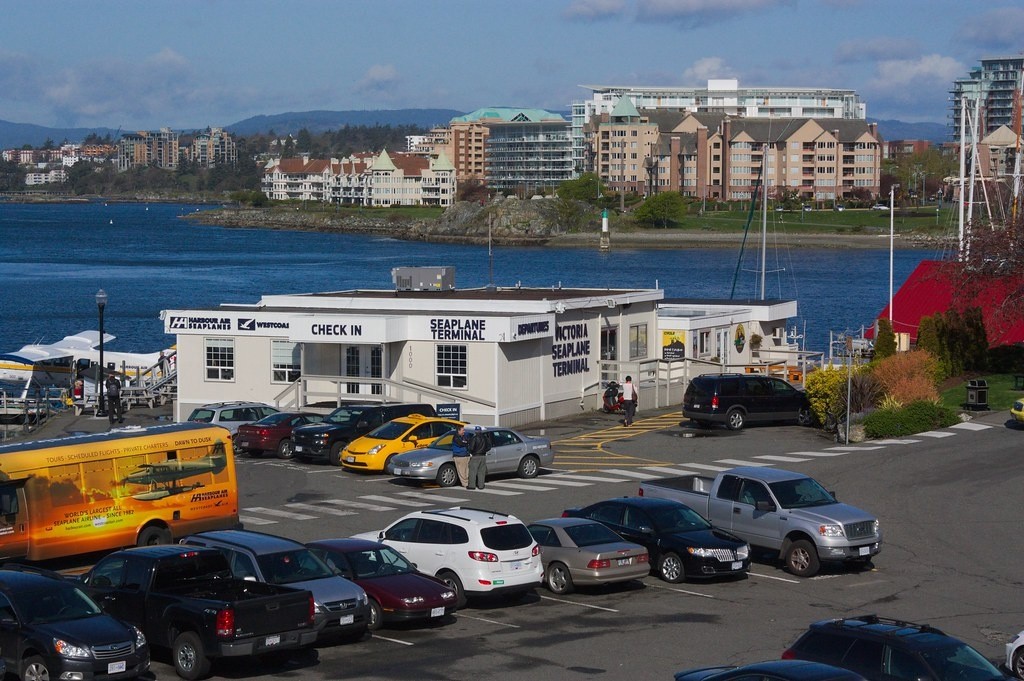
[602,381,638,414]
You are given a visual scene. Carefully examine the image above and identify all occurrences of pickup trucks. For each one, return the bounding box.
[638,465,883,578]
[62,544,319,681]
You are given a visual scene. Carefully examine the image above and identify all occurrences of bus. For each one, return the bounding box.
[0,421,243,563]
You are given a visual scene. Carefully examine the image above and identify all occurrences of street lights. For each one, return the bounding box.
[95,288,108,417]
[923,172,935,206]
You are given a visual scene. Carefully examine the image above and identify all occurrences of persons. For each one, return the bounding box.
[622,376,637,427]
[465,425,491,490]
[158,350,171,377]
[105,370,123,425]
[74,380,83,416]
[452,424,471,488]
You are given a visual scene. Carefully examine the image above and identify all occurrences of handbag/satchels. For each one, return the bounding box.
[632,384,637,401]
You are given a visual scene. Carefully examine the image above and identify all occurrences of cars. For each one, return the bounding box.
[235,410,337,460]
[835,204,845,212]
[673,658,869,681]
[340,414,470,476]
[775,205,784,212]
[561,496,752,584]
[872,203,890,211]
[929,195,936,201]
[523,517,652,595]
[387,423,554,488]
[803,205,812,212]
[285,538,459,633]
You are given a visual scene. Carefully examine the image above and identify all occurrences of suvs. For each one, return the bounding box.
[187,401,283,456]
[288,403,439,467]
[347,506,545,611]
[0,561,150,681]
[781,613,1024,681]
[180,528,370,643]
[682,373,817,432]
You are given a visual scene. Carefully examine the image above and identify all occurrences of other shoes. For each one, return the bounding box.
[628,425,632,427]
[624,420,627,426]
[479,484,485,489]
[110,421,113,423]
[466,486,475,490]
[117,419,123,423]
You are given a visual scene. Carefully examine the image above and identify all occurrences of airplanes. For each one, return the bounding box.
[122,438,227,501]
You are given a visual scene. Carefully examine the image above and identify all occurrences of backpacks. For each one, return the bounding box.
[107,380,119,397]
